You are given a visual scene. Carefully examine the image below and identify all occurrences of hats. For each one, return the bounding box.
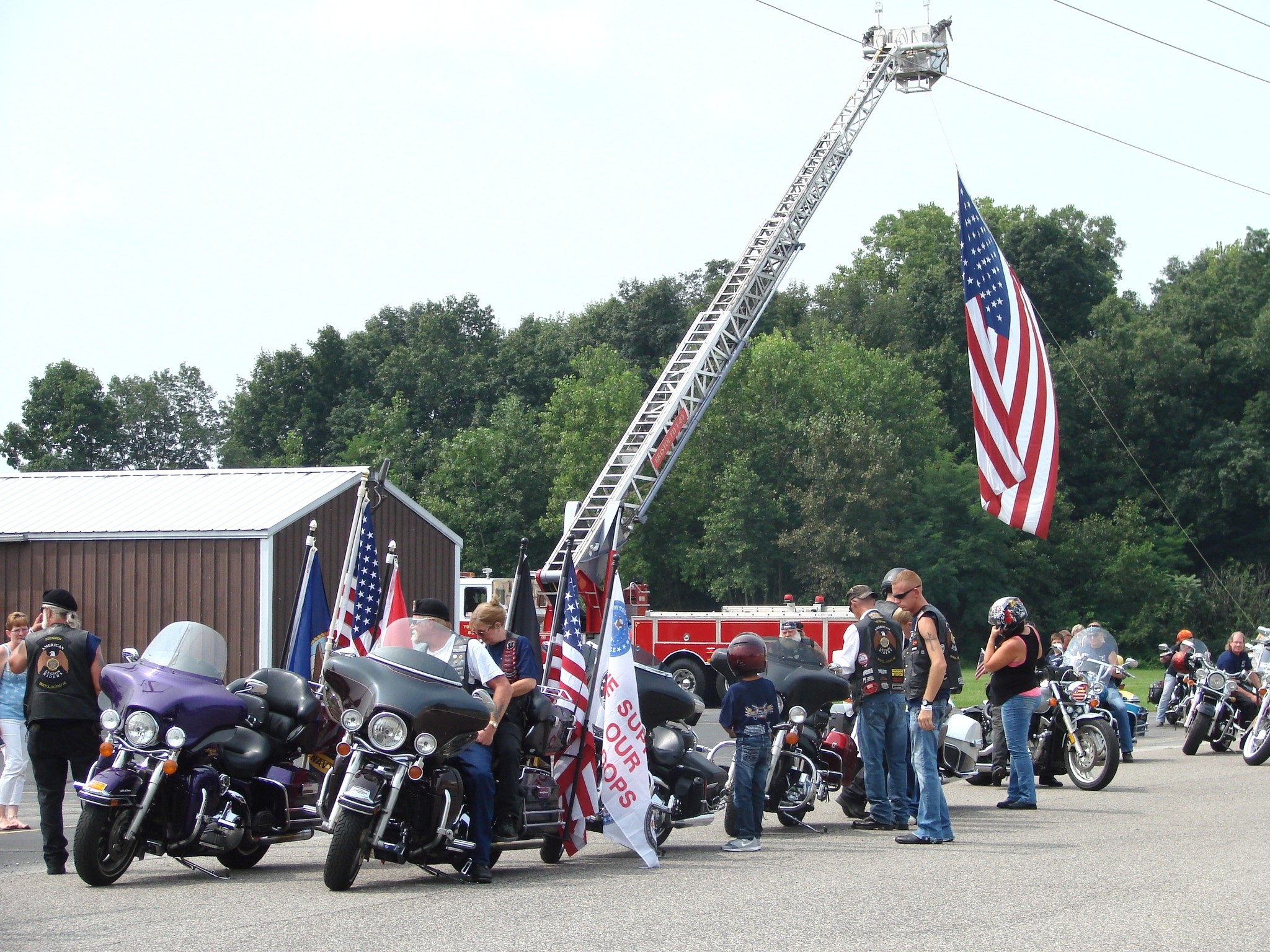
[846,585,878,605]
[42,589,78,611]
[412,599,450,622]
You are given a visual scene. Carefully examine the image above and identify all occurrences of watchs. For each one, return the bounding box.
[487,719,498,729]
[921,698,934,706]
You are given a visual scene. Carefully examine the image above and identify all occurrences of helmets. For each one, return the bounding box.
[881,568,907,598]
[988,597,1027,640]
[727,631,767,676]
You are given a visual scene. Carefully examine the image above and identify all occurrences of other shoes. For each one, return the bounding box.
[470,863,492,883]
[492,814,519,841]
[47,865,66,874]
[1007,801,1037,809]
[1123,751,1133,763]
[835,793,870,819]
[1039,775,1063,786]
[997,799,1014,809]
[1156,721,1164,726]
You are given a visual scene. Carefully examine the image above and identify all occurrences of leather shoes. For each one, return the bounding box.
[851,816,893,830]
[895,834,943,844]
[893,821,908,830]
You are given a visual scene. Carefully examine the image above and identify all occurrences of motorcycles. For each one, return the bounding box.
[303,616,1270,894]
[71,620,320,887]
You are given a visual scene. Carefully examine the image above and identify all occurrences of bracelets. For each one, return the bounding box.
[28,627,35,634]
[920,704,933,711]
[21,636,26,640]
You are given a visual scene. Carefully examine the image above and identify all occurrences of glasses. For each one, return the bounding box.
[412,618,435,625]
[892,585,921,599]
[40,606,46,613]
[471,625,494,636]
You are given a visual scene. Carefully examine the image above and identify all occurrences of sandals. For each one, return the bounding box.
[7,815,31,829]
[0,814,18,831]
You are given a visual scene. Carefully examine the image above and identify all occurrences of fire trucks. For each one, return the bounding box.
[457,1,956,709]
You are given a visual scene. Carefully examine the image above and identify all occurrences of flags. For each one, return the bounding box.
[285,492,412,724]
[541,552,659,869]
[510,555,545,687]
[954,166,1060,540]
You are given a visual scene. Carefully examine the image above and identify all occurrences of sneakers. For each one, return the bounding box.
[721,836,761,851]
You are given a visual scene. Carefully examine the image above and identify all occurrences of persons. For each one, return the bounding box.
[409,598,513,883]
[1153,629,1266,732]
[8,589,107,875]
[983,594,1135,787]
[0,611,33,830]
[719,632,780,851]
[834,568,921,830]
[769,619,831,669]
[891,570,964,844]
[983,597,1043,810]
[469,595,537,842]
